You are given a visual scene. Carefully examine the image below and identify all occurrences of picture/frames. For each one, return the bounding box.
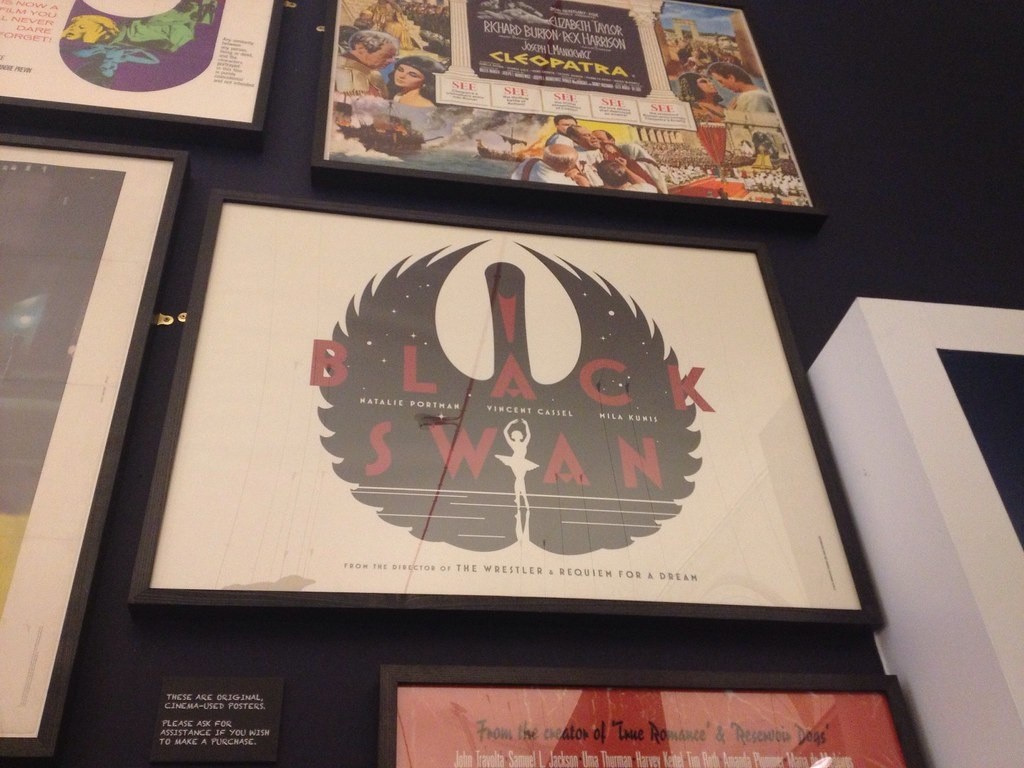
[128,188,888,639]
[377,663,928,768]
[310,0,826,242]
[0,0,285,137]
[0,138,193,765]
